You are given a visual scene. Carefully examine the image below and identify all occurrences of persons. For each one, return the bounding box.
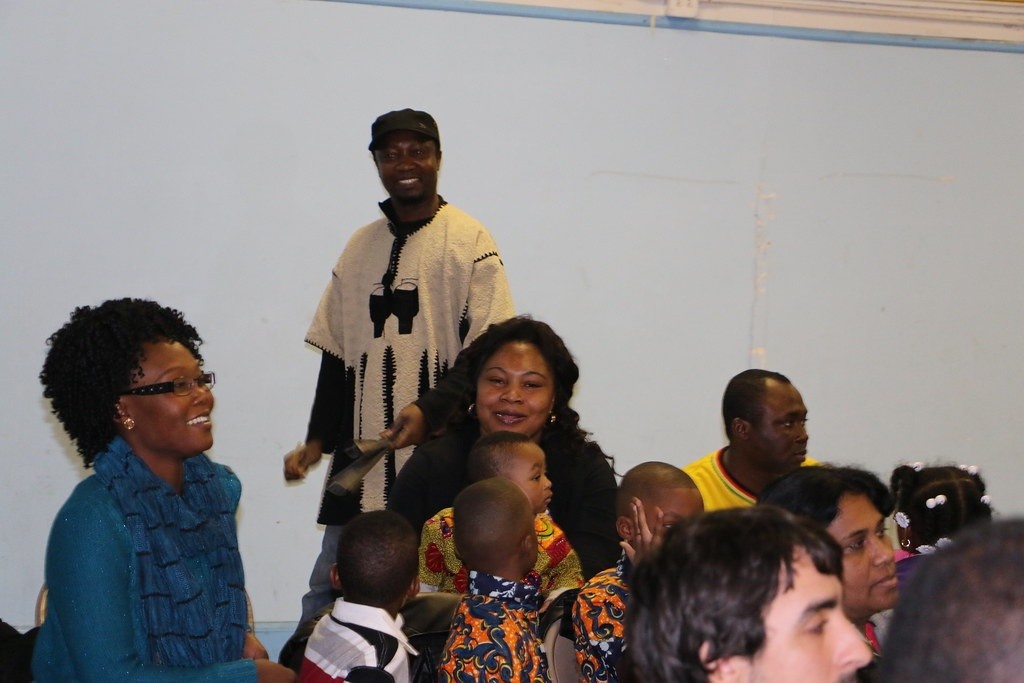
[757,465,900,660]
[624,507,872,683]
[890,465,992,560]
[284,111,516,619]
[571,462,704,683]
[872,517,1023,683]
[681,368,832,507]
[32,297,297,683]
[296,511,420,683]
[417,430,583,595]
[384,314,624,576]
[437,477,549,683]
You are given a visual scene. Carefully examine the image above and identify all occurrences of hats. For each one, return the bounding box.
[369,107,439,150]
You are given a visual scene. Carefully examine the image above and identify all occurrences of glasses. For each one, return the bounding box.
[121,370,216,397]
